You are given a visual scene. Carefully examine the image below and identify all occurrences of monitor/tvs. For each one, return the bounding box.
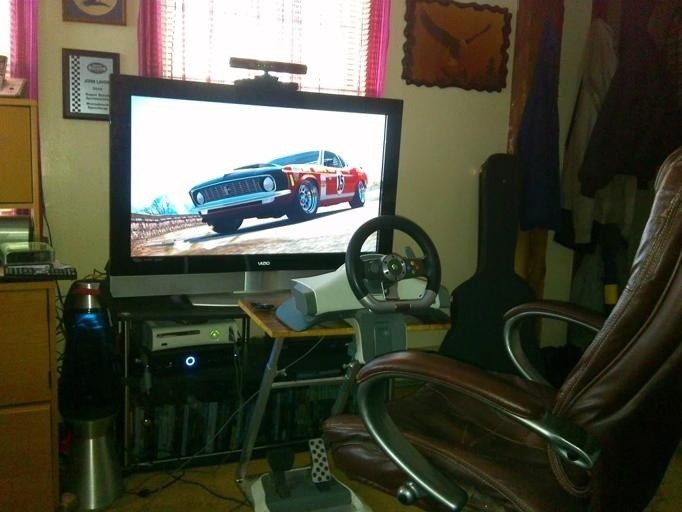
[108,72,404,308]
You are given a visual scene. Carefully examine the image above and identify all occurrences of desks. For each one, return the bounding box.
[112,291,364,474]
[236,294,452,482]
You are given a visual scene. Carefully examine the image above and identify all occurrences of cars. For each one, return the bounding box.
[190,148,367,235]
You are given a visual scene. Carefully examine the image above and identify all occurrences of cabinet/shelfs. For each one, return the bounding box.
[0,97,60,512]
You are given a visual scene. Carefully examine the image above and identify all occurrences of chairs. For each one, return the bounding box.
[318,145,680,511]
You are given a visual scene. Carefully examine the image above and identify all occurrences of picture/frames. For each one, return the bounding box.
[60,48,121,121]
[62,0,128,27]
